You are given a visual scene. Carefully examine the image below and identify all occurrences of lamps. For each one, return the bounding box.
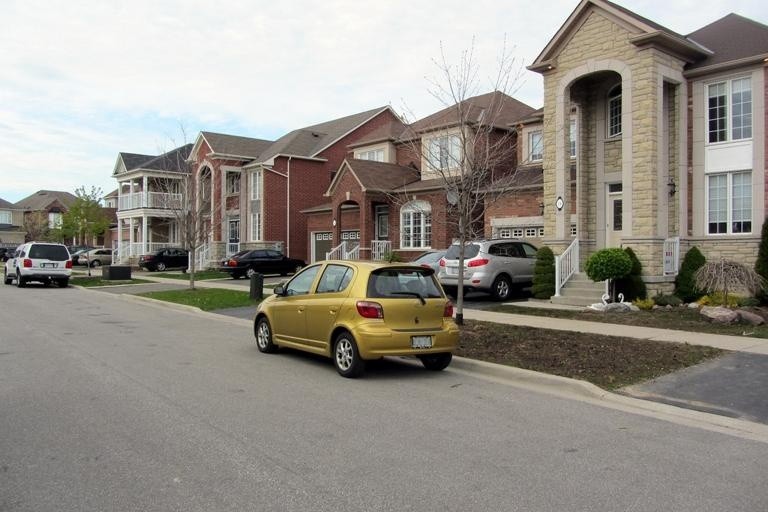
[538,201,544,214]
[345,191,350,201]
[666,176,677,197]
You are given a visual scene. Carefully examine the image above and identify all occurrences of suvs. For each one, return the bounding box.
[434,236,539,299]
[3,240,73,288]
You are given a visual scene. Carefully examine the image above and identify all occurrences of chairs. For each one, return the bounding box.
[314,276,328,292]
[406,280,424,294]
[330,274,348,292]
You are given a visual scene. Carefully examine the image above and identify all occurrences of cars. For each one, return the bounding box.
[137,247,189,272]
[0,244,112,268]
[218,248,306,280]
[398,249,447,287]
[250,258,464,379]
